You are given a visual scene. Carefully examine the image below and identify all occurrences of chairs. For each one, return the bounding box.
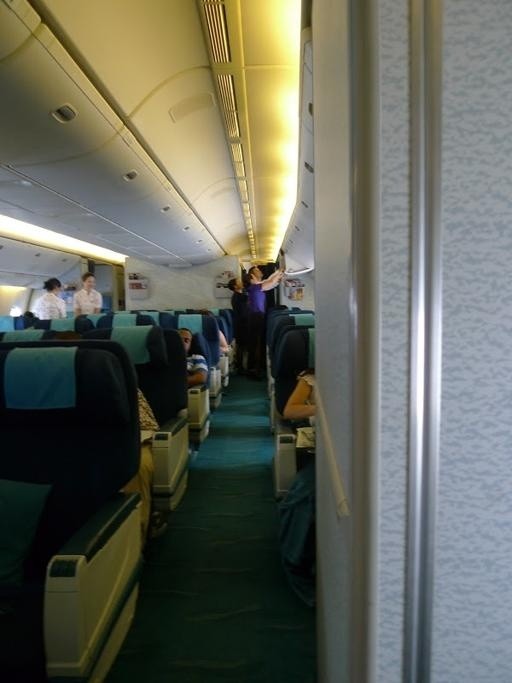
[263,305,316,501]
[1,308,237,542]
[0,338,143,682]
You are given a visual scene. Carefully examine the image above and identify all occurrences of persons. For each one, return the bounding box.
[203,310,229,354]
[283,366,316,427]
[52,332,81,340]
[73,271,103,315]
[119,389,161,548]
[33,279,67,320]
[227,265,284,380]
[177,326,208,386]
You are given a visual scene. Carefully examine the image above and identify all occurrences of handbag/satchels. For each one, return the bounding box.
[296,426,316,449]
